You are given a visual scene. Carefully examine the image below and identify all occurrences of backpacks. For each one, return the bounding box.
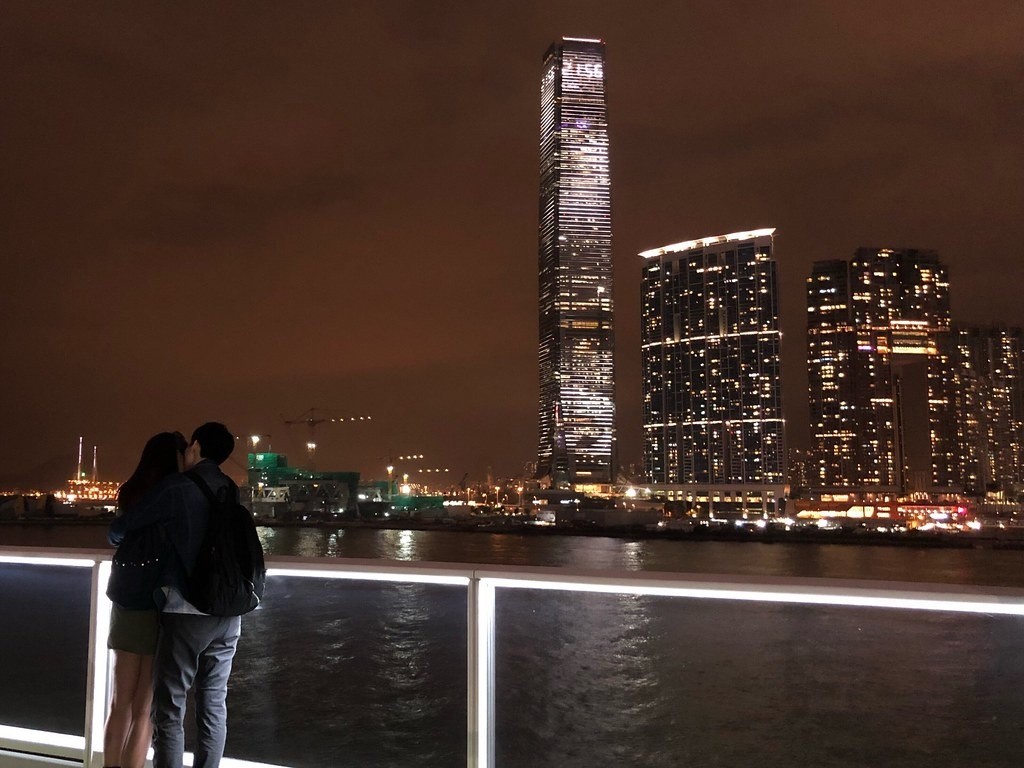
[108,498,175,611]
[167,473,265,617]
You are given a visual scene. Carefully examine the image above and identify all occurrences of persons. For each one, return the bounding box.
[103,419,268,768]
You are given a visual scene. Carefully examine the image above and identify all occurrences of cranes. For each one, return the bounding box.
[285,408,371,472]
